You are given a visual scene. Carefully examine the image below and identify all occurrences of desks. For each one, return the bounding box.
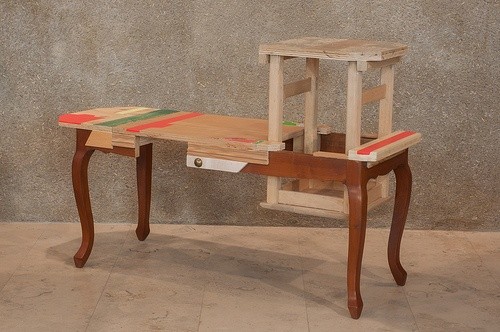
[58,106,421,320]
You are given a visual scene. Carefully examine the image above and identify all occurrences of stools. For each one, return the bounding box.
[255,37,409,220]
